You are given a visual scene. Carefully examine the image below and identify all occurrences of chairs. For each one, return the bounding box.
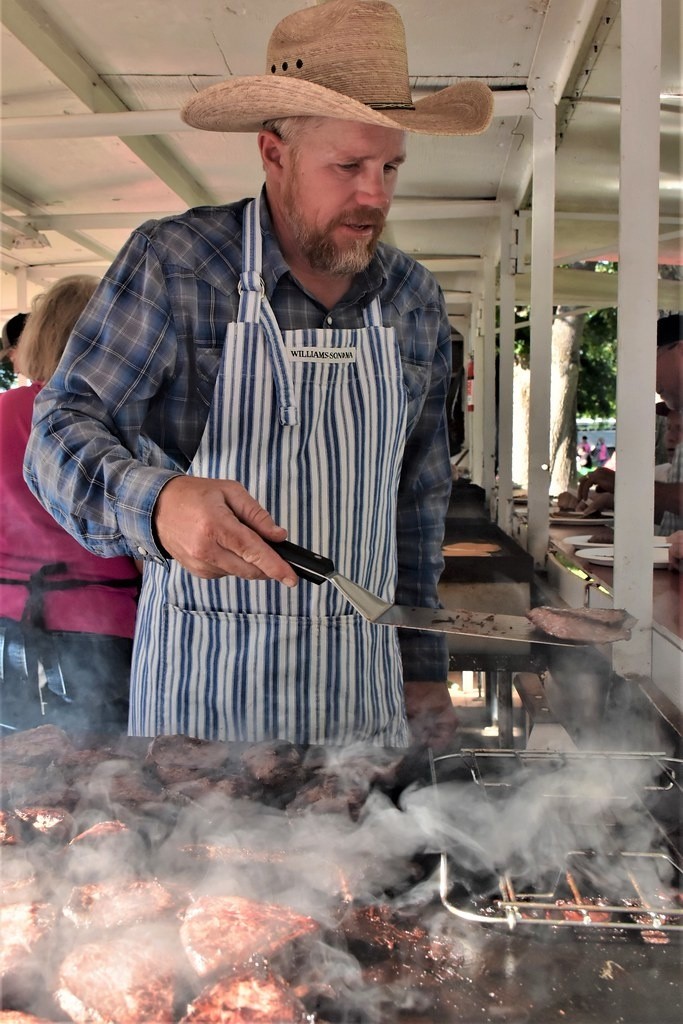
[445,478,541,756]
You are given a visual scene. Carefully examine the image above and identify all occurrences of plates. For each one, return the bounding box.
[515,507,561,516]
[548,511,615,527]
[562,532,672,552]
[575,544,673,572]
[510,496,554,504]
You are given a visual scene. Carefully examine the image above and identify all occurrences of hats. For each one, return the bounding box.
[180,1,494,138]
[0,313,30,361]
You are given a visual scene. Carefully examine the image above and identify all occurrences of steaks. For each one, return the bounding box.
[525,606,637,644]
[0,722,416,1024]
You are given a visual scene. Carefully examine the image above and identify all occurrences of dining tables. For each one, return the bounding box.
[510,496,682,640]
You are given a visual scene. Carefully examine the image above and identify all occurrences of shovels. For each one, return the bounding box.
[262,539,592,647]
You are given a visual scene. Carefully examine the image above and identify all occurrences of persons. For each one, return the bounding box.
[577,435,607,469]
[0,275,144,737]
[0,312,33,389]
[666,529,683,574]
[573,400,683,525]
[19,2,494,752]
[579,313,683,537]
